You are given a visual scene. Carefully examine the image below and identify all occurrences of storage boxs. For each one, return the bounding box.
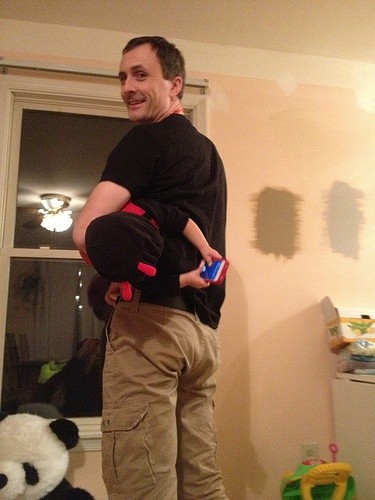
[320,297,375,353]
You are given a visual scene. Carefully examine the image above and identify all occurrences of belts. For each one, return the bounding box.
[117,287,200,315]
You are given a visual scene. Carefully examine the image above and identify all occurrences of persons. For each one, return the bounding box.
[38,281,115,419]
[84,198,223,303]
[71,35,229,500]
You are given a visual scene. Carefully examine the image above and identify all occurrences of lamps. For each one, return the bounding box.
[38,211,74,233]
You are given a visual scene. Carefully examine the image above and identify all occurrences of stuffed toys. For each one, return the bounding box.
[0,411,95,500]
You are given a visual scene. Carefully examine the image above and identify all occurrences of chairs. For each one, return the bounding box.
[6,332,49,396]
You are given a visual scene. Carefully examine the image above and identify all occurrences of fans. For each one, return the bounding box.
[18,193,80,230]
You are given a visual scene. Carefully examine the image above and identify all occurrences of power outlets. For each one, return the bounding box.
[301,442,318,461]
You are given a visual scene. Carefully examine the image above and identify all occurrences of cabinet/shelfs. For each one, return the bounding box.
[331,375,375,500]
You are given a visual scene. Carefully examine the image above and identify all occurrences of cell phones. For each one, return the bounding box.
[199,258,229,285]
